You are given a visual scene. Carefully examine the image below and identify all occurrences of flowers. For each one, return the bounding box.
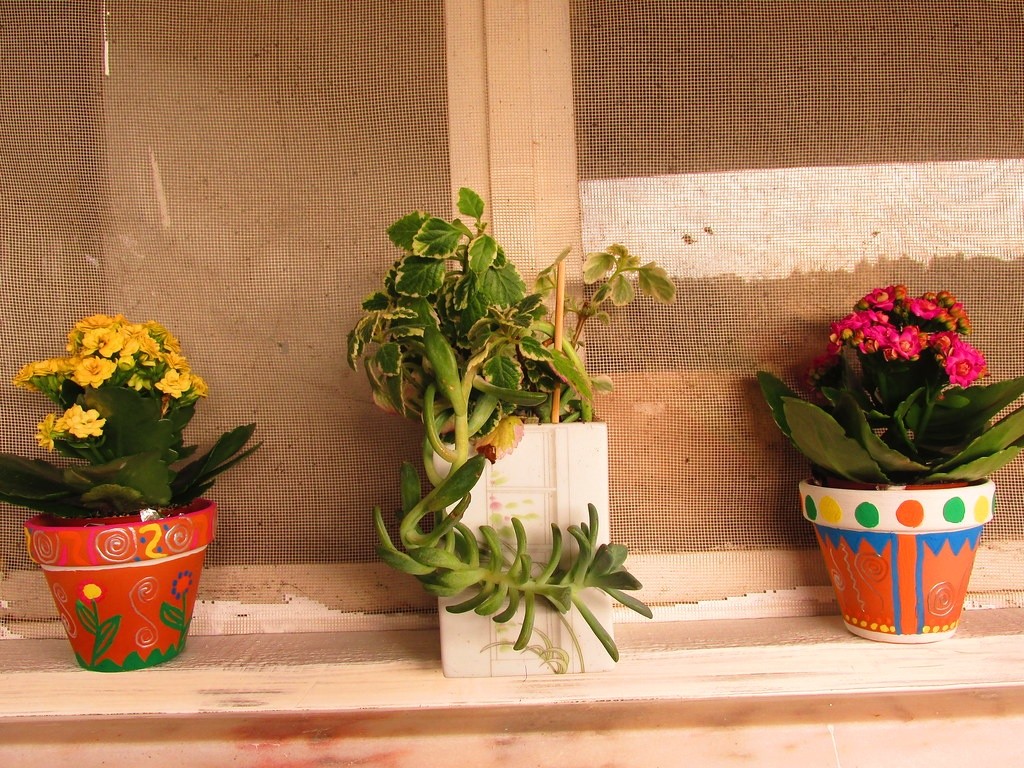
[0,313,265,512]
[756,283,1024,484]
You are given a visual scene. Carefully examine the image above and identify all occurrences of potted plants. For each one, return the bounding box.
[347,187,681,680]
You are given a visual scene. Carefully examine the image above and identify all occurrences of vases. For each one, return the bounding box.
[22,501,219,672]
[798,477,999,644]
